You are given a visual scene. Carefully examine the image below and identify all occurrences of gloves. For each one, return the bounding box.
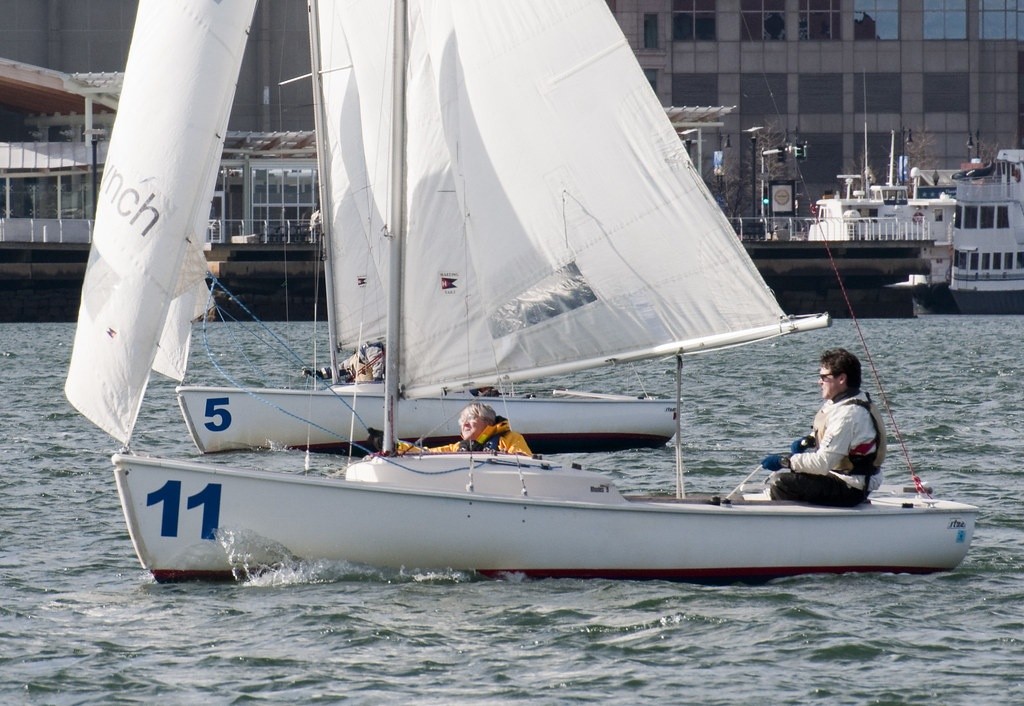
[790,435,813,455]
[365,427,399,452]
[302,366,323,381]
[760,454,783,471]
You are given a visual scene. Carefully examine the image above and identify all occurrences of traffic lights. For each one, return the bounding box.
[763,197,770,208]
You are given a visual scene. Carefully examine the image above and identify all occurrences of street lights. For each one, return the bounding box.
[967,132,974,163]
[719,131,733,193]
[901,126,914,185]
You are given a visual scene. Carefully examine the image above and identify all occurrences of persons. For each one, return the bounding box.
[396,403,532,458]
[761,347,887,508]
[748,219,766,240]
[843,206,861,240]
[302,342,385,383]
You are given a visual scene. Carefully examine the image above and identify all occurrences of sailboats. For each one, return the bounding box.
[64,0,981,583]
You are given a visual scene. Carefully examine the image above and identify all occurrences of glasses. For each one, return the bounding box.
[819,373,833,382]
